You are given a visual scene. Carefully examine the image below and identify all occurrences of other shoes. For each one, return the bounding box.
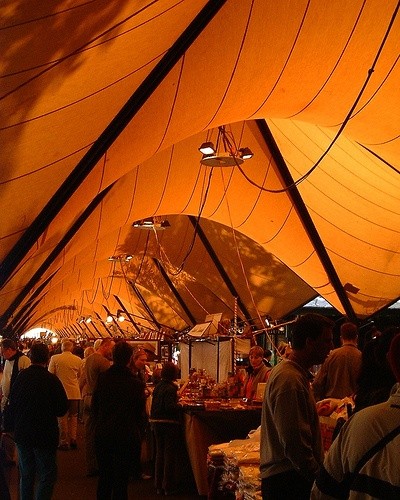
[57,442,70,449]
[87,470,98,477]
[130,475,151,483]
[70,439,76,448]
[156,488,164,495]
[165,488,184,496]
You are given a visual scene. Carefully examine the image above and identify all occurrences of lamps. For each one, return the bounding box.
[85,315,102,323]
[108,217,171,261]
[197,142,217,159]
[106,313,173,339]
[117,310,178,332]
[239,147,254,160]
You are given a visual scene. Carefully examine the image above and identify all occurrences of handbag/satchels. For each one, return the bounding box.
[3,403,14,431]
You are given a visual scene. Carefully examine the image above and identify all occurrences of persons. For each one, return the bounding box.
[0,334,126,424]
[76,338,116,479]
[312,323,362,400]
[0,337,32,406]
[311,332,400,500]
[262,349,272,367]
[88,342,148,500]
[255,313,334,500]
[146,362,186,496]
[47,341,84,450]
[352,338,392,414]
[3,343,70,500]
[244,345,271,400]
[263,349,273,367]
[125,349,157,480]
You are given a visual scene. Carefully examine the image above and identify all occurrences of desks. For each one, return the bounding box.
[180,395,263,500]
